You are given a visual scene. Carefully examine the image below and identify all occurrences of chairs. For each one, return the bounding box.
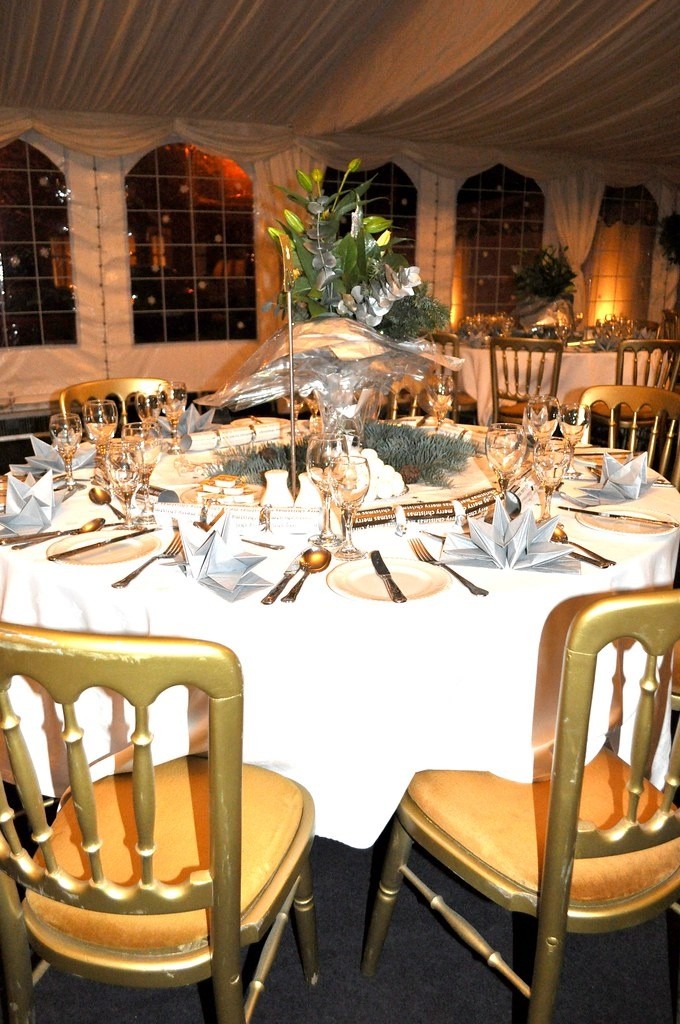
[59,378,173,443]
[362,588,680,1024]
[0,621,316,1024]
[389,312,680,493]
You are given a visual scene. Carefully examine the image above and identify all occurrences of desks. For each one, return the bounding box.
[427,340,673,429]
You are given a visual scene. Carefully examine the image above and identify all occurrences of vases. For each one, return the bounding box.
[316,386,372,457]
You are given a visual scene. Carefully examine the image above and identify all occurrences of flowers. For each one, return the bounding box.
[511,241,577,301]
[266,157,450,344]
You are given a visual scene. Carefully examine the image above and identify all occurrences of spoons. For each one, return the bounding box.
[585,467,675,487]
[193,522,285,550]
[551,526,617,566]
[280,546,332,604]
[11,518,106,551]
[89,487,126,519]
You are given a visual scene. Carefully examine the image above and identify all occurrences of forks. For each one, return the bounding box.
[111,533,184,590]
[408,538,489,598]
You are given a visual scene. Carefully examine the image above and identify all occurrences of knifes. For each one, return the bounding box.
[47,529,156,562]
[417,414,431,426]
[557,506,680,529]
[0,522,125,546]
[573,451,644,457]
[370,550,408,603]
[261,547,313,605]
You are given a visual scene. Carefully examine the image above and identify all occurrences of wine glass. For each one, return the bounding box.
[302,393,319,435]
[426,375,456,435]
[157,381,188,456]
[136,390,161,439]
[485,423,527,523]
[83,399,119,485]
[305,433,350,549]
[105,438,145,531]
[283,395,305,438]
[558,402,591,479]
[327,455,371,562]
[121,422,162,526]
[533,437,572,530]
[522,395,561,472]
[49,412,87,493]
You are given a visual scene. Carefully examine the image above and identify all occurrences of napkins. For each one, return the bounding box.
[589,334,622,352]
[575,452,659,505]
[9,436,97,476]
[185,514,274,604]
[0,468,65,536]
[152,403,215,439]
[440,494,582,575]
[632,327,655,339]
[466,331,484,348]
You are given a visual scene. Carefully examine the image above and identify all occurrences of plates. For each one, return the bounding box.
[326,556,453,605]
[575,505,680,538]
[574,447,631,468]
[180,483,265,506]
[398,416,455,430]
[45,529,162,568]
[231,417,292,430]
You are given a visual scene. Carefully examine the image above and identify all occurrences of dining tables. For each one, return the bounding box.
[0,419,680,850]
